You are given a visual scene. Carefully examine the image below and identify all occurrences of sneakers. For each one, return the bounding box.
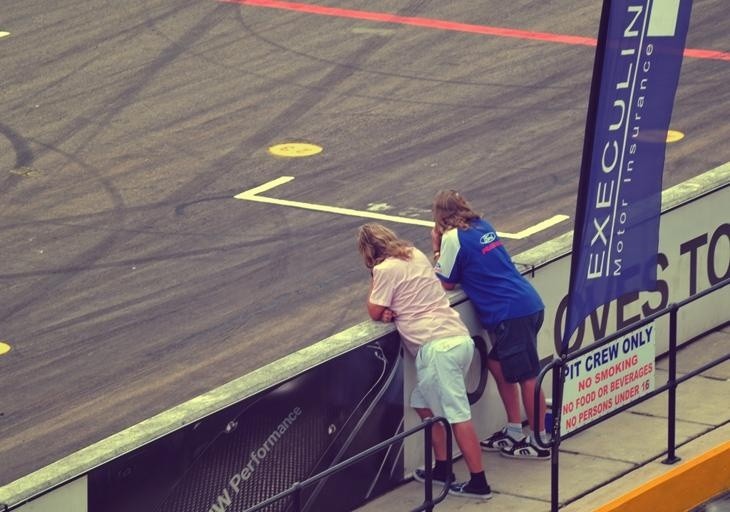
[478,430,525,452]
[412,468,456,485]
[447,482,491,500]
[502,438,552,462]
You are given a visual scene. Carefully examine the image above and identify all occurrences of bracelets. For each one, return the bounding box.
[433,250,440,259]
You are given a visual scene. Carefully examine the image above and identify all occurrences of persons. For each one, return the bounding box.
[356,222,493,500]
[430,189,550,460]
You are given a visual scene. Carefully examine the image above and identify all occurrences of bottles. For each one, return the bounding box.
[545,398,553,438]
